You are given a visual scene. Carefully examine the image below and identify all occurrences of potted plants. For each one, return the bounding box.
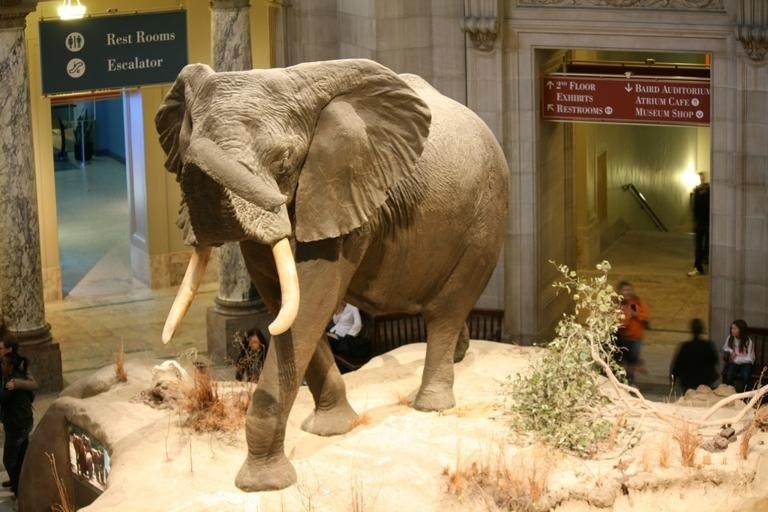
[72,106,97,161]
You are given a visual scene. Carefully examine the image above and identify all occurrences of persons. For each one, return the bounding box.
[669,318,721,395]
[613,281,649,385]
[328,299,363,374]
[687,172,710,277]
[235,328,268,383]
[721,318,756,393]
[0,351,37,499]
[0,332,39,492]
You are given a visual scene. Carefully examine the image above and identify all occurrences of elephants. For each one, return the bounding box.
[70,432,111,486]
[154,58,513,493]
